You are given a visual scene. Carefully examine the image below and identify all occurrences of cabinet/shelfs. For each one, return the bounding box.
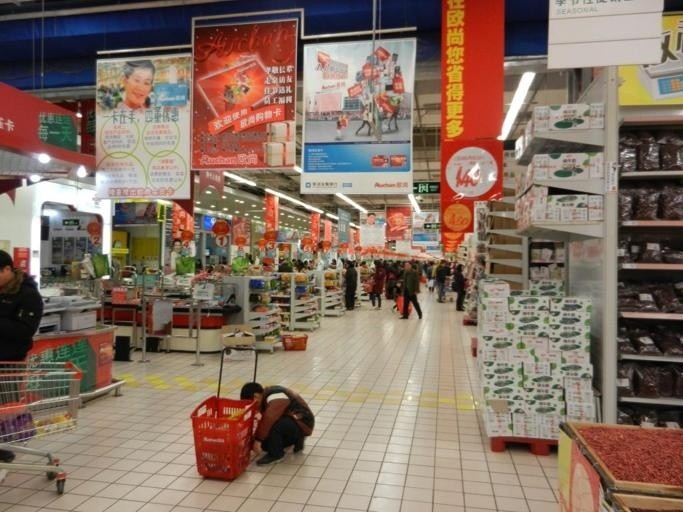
[222,268,368,338]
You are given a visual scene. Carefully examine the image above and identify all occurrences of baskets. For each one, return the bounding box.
[190,396,258,481]
[282,333,308,350]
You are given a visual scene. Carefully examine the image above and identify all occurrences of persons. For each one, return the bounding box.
[2,248,44,466]
[195,255,470,320]
[169,239,183,272]
[239,380,315,467]
[96,59,158,111]
[331,46,407,143]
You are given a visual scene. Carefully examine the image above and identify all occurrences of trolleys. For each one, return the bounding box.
[0,360,83,495]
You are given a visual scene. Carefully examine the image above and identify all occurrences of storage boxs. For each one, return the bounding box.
[480,412,598,439]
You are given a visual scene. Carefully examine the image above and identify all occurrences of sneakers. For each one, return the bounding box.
[456,305,465,311]
[399,313,422,320]
[256,452,285,466]
[293,437,304,452]
[1,450,15,463]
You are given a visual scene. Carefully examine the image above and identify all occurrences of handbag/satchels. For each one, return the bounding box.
[452,281,457,292]
